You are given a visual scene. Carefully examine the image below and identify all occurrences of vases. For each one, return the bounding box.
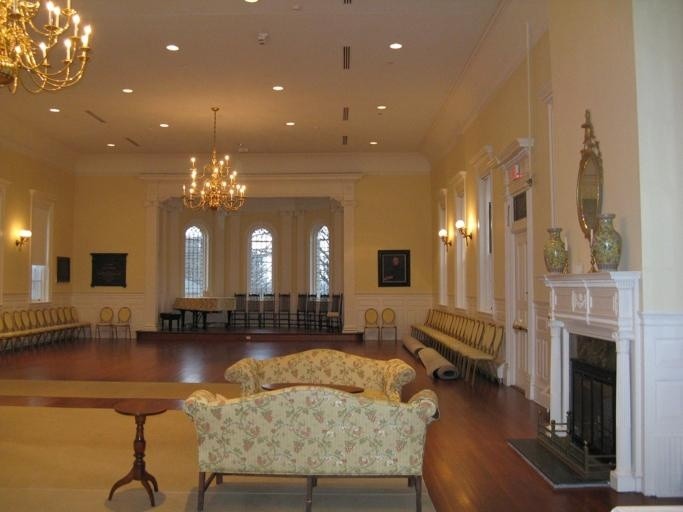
[543,227,569,276]
[591,213,623,271]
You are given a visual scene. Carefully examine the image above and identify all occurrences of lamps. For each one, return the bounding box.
[0,0,93,95]
[181,107,248,212]
[438,229,452,253]
[15,230,32,249]
[455,220,473,247]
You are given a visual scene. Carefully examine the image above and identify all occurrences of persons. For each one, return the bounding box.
[384,255,404,281]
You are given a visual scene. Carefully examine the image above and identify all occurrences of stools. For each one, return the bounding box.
[159,311,182,332]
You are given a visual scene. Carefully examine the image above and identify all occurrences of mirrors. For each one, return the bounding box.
[574,109,603,241]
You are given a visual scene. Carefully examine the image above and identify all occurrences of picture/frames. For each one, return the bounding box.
[378,249,410,288]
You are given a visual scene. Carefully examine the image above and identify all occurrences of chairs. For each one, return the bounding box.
[114,306,132,343]
[0,306,92,362]
[362,307,381,344]
[95,307,116,344]
[410,303,505,389]
[227,291,344,335]
[379,307,398,344]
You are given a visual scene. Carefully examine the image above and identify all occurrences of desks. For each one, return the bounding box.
[107,399,167,508]
[172,297,237,333]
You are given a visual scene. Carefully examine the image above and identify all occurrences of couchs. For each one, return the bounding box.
[225,348,418,409]
[183,387,440,512]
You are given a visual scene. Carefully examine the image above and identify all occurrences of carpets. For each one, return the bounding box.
[1,376,435,512]
